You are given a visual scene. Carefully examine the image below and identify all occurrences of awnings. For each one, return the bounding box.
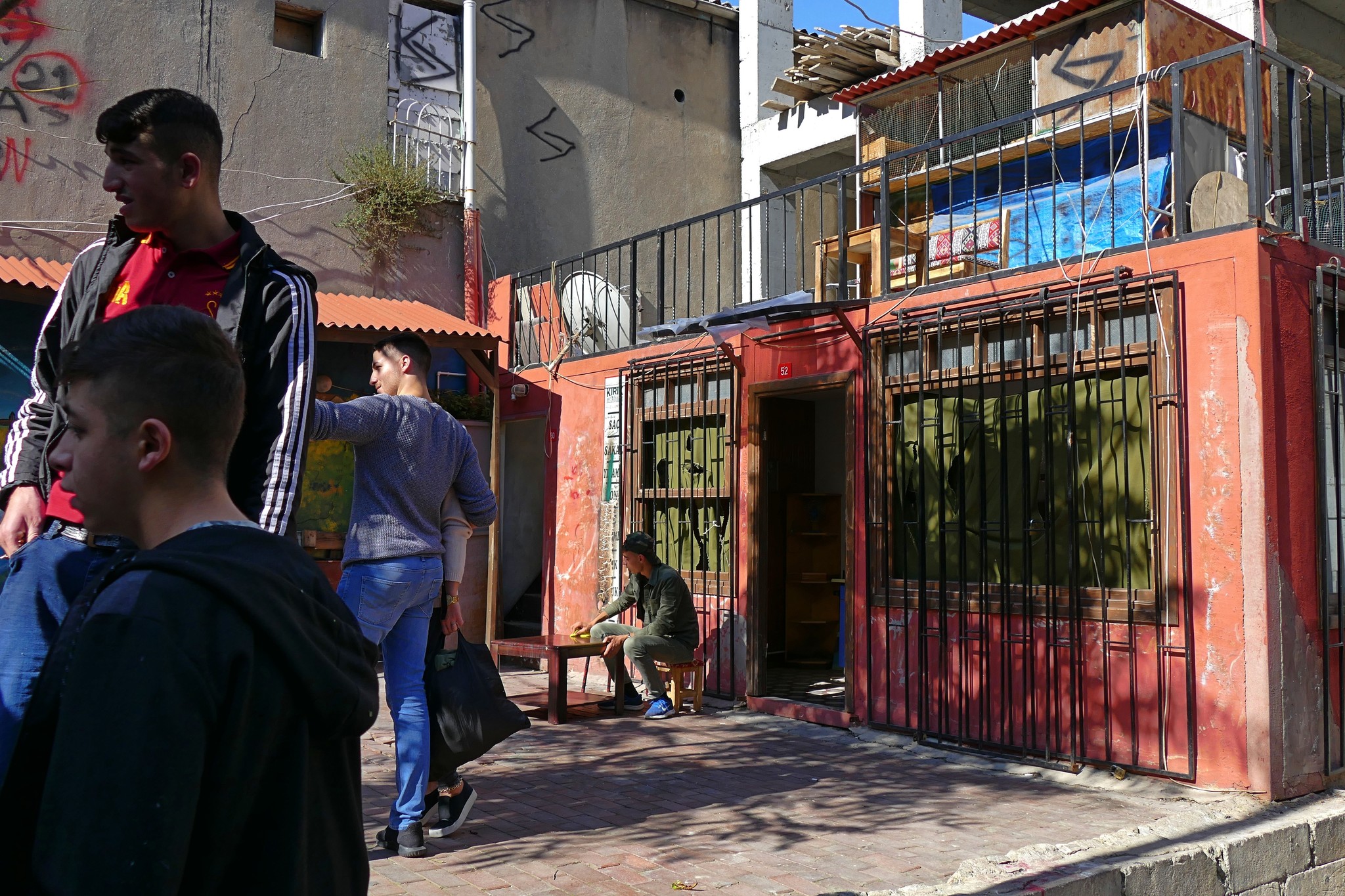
[1,255,510,649]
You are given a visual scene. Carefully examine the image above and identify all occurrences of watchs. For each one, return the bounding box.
[446,595,459,606]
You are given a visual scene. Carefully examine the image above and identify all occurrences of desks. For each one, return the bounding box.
[812,223,931,304]
[489,633,624,725]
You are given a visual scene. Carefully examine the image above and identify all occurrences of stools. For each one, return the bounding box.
[653,659,705,715]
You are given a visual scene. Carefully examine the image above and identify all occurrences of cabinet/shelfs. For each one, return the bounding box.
[782,491,841,671]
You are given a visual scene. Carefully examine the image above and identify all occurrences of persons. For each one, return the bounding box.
[570,530,699,719]
[1,305,378,896]
[310,333,495,857]
[0,87,318,780]
[421,484,478,838]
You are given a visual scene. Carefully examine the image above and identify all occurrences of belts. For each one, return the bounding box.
[44,517,135,550]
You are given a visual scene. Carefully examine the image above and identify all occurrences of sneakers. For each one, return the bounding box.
[419,774,478,838]
[597,694,644,711]
[644,697,676,719]
[376,821,427,857]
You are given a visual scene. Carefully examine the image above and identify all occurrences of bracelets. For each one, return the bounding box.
[627,632,631,638]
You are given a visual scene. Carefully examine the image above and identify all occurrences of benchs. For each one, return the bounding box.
[854,208,1011,298]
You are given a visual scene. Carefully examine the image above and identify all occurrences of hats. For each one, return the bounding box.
[623,531,661,565]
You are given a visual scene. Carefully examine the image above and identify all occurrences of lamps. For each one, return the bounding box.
[511,383,530,397]
